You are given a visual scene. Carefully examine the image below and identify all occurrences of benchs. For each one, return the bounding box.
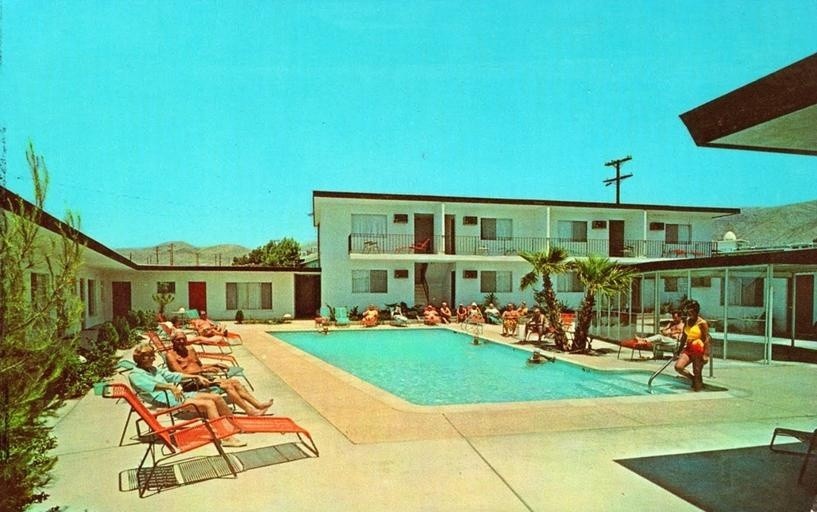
[543,313,575,340]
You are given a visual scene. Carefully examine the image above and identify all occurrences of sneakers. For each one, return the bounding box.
[222,439,247,447]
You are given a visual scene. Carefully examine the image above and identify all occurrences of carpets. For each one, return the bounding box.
[614,441,817,512]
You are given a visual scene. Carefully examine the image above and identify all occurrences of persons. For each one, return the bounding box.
[165,328,274,416]
[196,310,228,336]
[170,315,227,346]
[127,342,255,447]
[634,310,687,352]
[674,300,711,392]
[390,301,549,346]
[362,304,379,321]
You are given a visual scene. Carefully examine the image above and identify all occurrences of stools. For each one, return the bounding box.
[617,340,652,360]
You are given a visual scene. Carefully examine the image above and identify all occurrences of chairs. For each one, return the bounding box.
[333,304,500,326]
[97,321,319,498]
[408,237,430,254]
